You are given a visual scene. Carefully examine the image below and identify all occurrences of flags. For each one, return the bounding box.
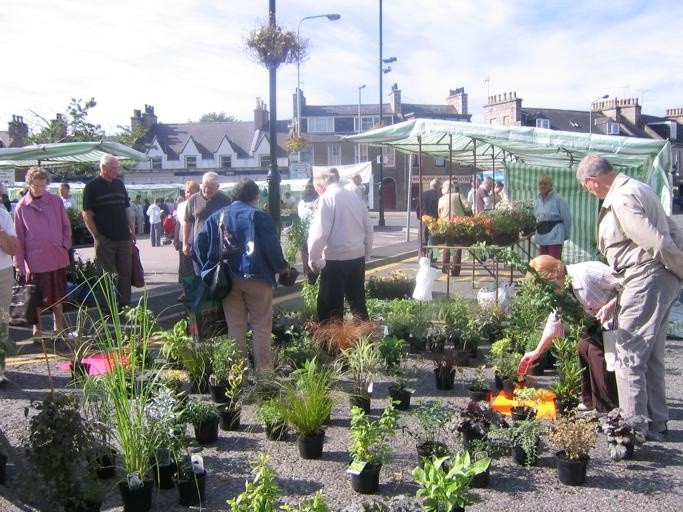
[430,226,535,246]
[258,45,289,64]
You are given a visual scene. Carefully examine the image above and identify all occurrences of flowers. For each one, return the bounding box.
[418,197,537,237]
[242,22,306,66]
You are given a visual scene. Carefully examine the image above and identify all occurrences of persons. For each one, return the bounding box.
[438,180,474,276]
[516,255,624,412]
[533,175,570,261]
[344,174,363,200]
[126,189,186,247]
[2,169,18,385]
[194,178,290,387]
[416,180,443,254]
[4,190,12,212]
[298,178,320,285]
[82,153,137,330]
[328,168,340,183]
[176,180,200,317]
[13,165,73,344]
[574,153,682,443]
[263,192,296,226]
[174,196,185,302]
[182,171,232,342]
[60,184,77,209]
[467,175,504,261]
[308,170,375,371]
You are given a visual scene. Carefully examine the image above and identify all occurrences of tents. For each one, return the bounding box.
[341,119,673,265]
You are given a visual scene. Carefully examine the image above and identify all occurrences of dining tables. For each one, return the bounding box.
[279,207,312,286]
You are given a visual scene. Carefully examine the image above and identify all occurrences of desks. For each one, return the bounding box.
[423,232,536,306]
[588,94,608,134]
[358,85,367,163]
[296,13,341,163]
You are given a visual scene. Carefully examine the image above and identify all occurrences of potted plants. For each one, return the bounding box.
[279,207,312,286]
[0,241,603,511]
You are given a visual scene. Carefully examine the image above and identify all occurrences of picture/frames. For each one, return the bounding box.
[423,232,536,306]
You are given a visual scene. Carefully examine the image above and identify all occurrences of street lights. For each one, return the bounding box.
[10,274,38,326]
[132,241,145,286]
[209,264,231,300]
[533,220,563,235]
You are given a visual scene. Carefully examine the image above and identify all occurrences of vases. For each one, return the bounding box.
[258,45,289,64]
[418,197,537,237]
[430,226,535,246]
[242,22,306,66]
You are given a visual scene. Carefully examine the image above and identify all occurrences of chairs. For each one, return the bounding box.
[577,401,667,436]
[1,366,9,382]
